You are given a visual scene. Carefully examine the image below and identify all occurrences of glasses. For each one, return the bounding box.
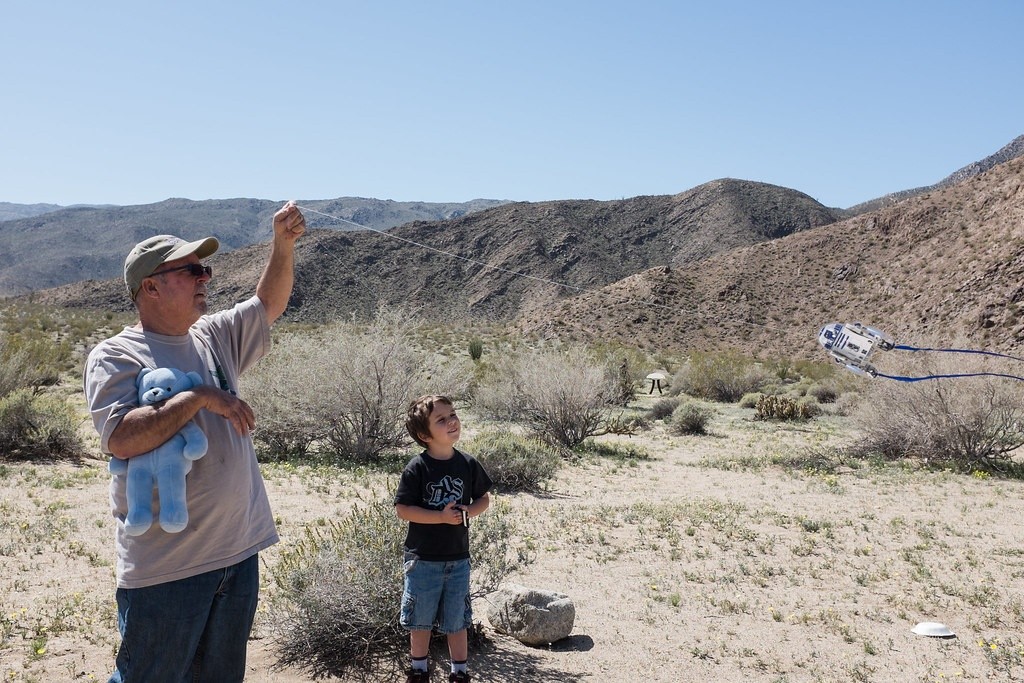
[148,264,212,278]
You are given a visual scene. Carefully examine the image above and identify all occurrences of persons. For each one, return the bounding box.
[394,394,493,683]
[83,201,302,683]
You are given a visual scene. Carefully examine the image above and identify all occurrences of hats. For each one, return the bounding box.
[124,235,219,299]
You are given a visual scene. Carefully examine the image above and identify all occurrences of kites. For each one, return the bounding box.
[818,322,1024,393]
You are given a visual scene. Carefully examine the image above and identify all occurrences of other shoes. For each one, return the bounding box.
[404,667,430,683]
[449,670,471,683]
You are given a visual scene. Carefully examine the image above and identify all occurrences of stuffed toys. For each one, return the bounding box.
[109,367,207,535]
